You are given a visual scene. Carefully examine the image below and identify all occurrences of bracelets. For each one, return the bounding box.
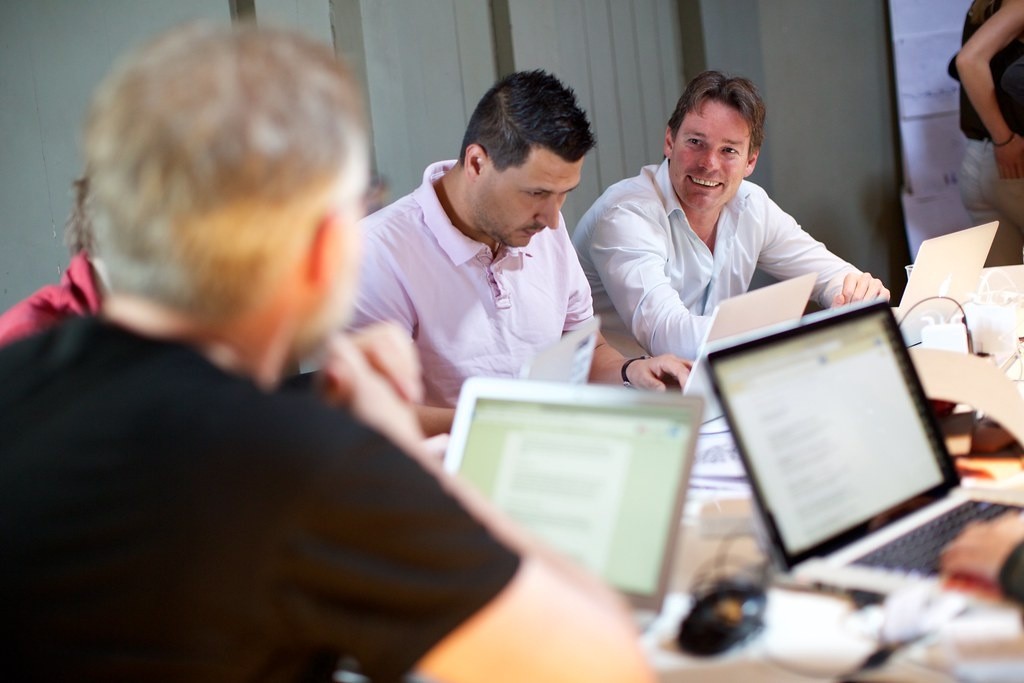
[991,131,1014,147]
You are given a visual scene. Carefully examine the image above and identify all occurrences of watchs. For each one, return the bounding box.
[621,355,654,389]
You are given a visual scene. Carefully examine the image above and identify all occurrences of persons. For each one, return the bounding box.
[0,26,668,682]
[339,69,694,438]
[948,1,1024,267]
[0,178,102,349]
[571,71,890,364]
[942,507,1024,604]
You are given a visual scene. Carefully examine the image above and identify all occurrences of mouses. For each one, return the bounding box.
[672,582,769,656]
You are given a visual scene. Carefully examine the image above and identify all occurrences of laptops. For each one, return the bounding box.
[446,220,1024,647]
